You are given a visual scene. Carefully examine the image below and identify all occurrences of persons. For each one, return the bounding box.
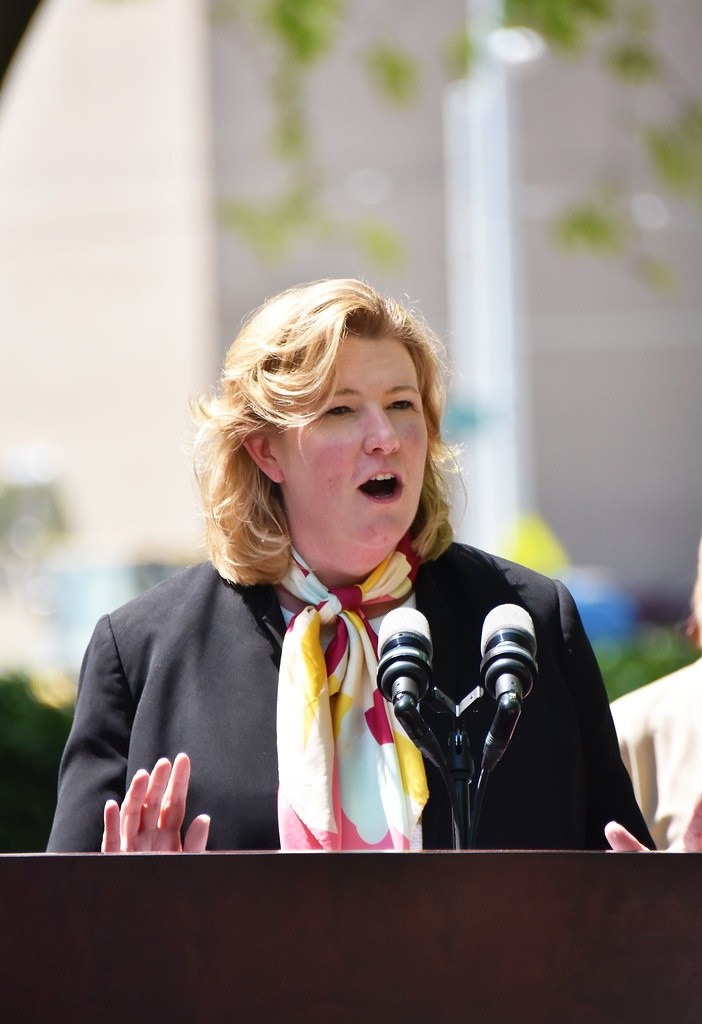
[608,537,702,853]
[47,279,702,853]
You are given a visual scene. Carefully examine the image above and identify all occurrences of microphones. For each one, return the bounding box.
[480,603,539,703]
[376,606,432,707]
